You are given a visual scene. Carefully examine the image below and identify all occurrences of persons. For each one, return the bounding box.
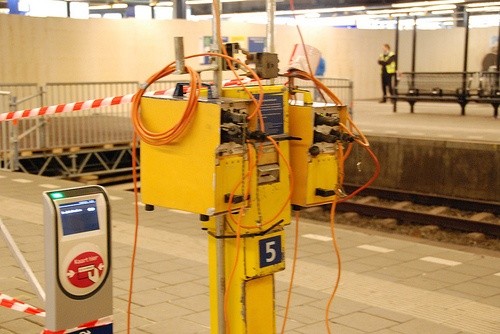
[482,47,497,72]
[378,44,398,103]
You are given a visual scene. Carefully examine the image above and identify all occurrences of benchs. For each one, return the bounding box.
[383,94,500,117]
